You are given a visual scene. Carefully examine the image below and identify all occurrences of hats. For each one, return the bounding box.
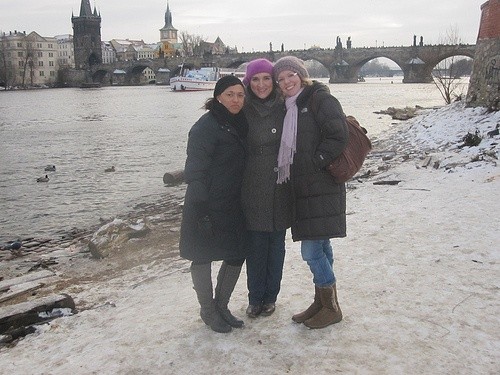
[243,59,273,86]
[272,56,309,83]
[214,76,243,97]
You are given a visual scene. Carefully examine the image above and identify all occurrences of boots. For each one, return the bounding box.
[292,285,320,322]
[190,262,232,333]
[303,281,341,329]
[215,264,244,328]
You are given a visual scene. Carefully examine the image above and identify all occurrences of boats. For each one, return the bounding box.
[169,66,247,91]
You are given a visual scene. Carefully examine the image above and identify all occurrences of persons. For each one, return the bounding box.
[273,57,348,328]
[179,76,251,333]
[239,57,299,317]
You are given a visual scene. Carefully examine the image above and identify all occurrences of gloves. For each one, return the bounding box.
[201,217,215,245]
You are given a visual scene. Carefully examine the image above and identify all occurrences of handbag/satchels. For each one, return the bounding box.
[324,114,372,184]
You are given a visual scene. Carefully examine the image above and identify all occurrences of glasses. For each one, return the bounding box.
[276,72,298,84]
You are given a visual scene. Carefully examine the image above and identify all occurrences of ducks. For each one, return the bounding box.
[43,165,56,172]
[103,165,116,172]
[36,174,50,182]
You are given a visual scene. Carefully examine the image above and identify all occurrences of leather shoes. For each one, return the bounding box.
[246,306,262,317]
[261,302,275,315]
